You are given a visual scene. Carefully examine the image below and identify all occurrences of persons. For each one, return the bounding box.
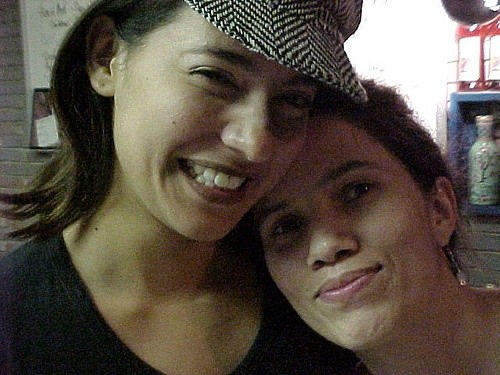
[0,0,367,375]
[253,80,500,375]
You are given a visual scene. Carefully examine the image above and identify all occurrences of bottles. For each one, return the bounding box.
[468,115,500,205]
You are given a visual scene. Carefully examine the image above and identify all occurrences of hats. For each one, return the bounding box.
[184,0,368,102]
